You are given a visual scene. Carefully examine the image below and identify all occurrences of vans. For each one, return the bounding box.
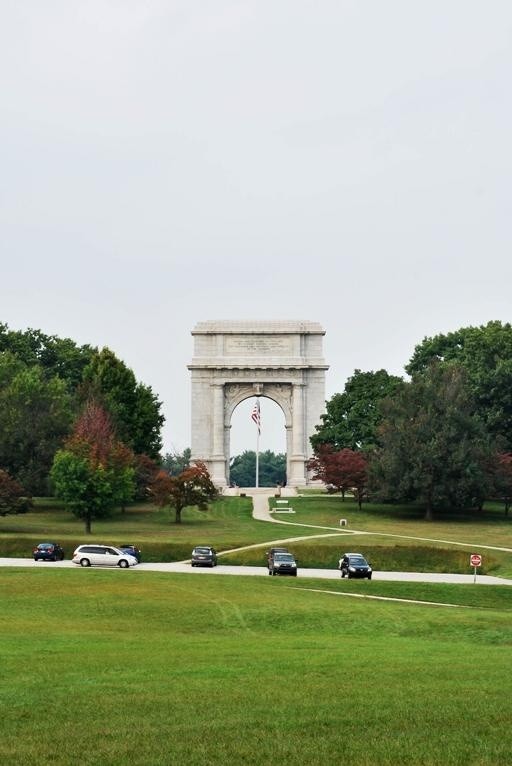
[70,545,139,569]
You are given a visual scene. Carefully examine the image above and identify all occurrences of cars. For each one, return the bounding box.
[268,553,299,578]
[265,547,289,569]
[342,556,372,581]
[192,547,218,568]
[120,544,141,563]
[337,553,363,569]
[33,542,66,562]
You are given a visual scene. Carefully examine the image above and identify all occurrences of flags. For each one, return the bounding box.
[251,400,260,435]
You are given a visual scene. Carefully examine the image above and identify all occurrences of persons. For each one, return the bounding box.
[275,480,282,497]
[282,480,286,487]
